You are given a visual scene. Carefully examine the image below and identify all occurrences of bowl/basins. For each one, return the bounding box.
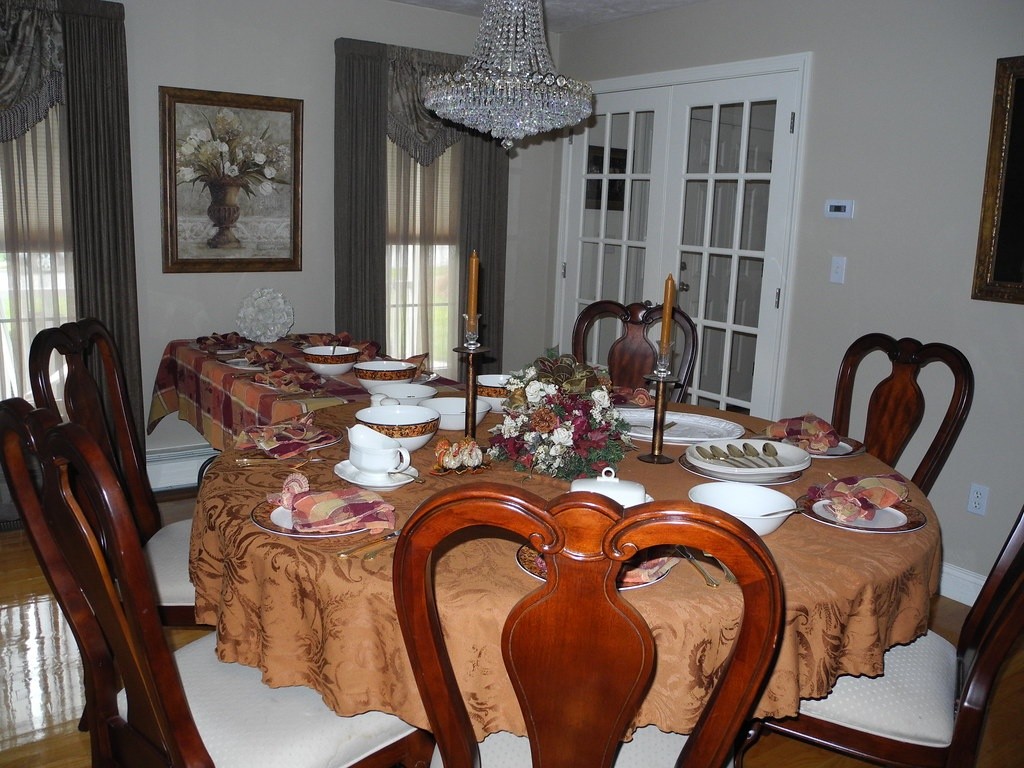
[302,346,360,378]
[475,374,512,414]
[353,360,417,389]
[355,405,441,454]
[418,397,492,431]
[368,383,439,405]
[688,482,797,537]
[685,438,812,481]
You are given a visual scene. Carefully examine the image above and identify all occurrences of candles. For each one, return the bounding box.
[468,249,479,334]
[660,275,676,346]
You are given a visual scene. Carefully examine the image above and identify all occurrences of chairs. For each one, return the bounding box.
[832,333,974,500]
[0,396,441,768]
[735,507,1023,768]
[30,315,232,735]
[572,300,699,403]
[393,484,790,768]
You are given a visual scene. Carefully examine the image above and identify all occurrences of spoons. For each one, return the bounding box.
[763,443,785,467]
[710,445,755,468]
[727,444,764,468]
[743,443,776,467]
[696,446,744,468]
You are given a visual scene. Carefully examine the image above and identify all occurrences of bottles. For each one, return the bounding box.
[369,394,388,407]
[380,397,400,406]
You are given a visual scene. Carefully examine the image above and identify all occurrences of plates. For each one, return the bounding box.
[188,342,441,391]
[678,452,803,485]
[810,436,867,459]
[795,494,927,533]
[614,407,746,446]
[250,499,371,538]
[516,540,671,591]
[306,423,343,452]
[334,459,419,492]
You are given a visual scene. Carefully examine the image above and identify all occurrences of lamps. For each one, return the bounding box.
[423,0,593,152]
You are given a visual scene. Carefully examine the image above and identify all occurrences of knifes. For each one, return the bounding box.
[235,457,328,462]
[337,529,401,558]
[675,544,720,588]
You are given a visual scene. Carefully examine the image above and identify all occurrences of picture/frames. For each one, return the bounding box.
[586,144,635,212]
[160,86,307,275]
[970,56,1024,305]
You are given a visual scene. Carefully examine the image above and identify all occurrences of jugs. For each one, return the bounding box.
[346,423,410,484]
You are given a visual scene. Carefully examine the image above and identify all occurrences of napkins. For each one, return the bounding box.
[598,374,651,403]
[232,420,334,459]
[283,482,393,535]
[763,413,840,450]
[822,472,908,524]
[199,310,426,393]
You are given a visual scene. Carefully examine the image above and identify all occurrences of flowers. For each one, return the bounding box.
[487,343,638,485]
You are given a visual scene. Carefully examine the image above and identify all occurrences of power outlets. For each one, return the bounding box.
[970,487,988,514]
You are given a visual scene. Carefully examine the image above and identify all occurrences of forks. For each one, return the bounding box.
[630,422,677,432]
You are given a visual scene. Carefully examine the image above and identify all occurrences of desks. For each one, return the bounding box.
[146,334,457,454]
[186,392,916,743]
